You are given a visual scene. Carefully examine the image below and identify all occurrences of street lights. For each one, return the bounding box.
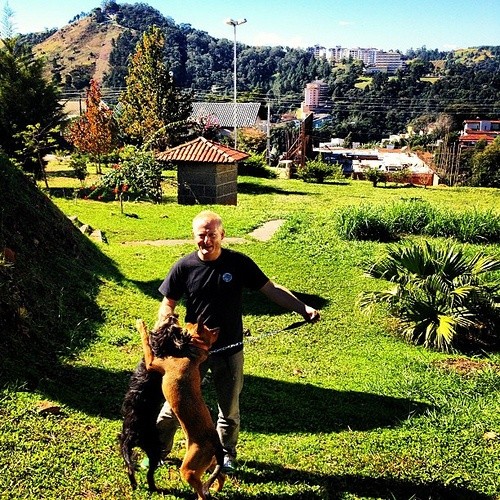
[226,17,247,150]
[265,102,272,167]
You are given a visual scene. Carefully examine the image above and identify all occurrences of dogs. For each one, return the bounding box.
[118,313,227,500]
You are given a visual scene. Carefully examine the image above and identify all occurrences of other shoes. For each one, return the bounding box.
[141,456,164,470]
[223,450,238,473]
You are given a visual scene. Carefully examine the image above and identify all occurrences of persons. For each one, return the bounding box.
[151,211,320,474]
